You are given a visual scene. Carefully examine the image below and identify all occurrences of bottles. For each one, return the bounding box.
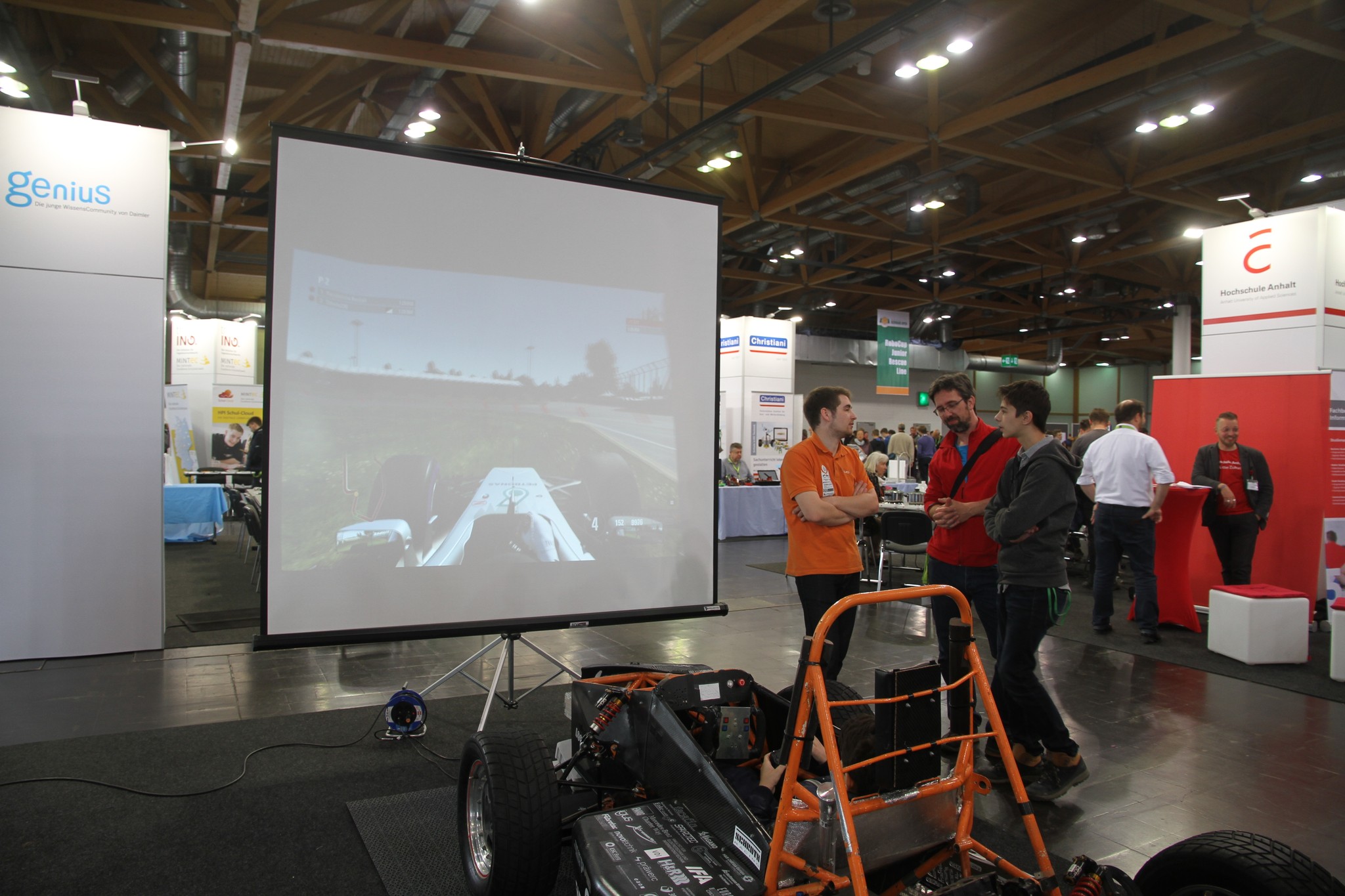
[915,484,925,492]
[921,481,928,491]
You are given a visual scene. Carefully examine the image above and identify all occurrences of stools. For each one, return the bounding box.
[1330,598,1345,681]
[1207,584,1310,667]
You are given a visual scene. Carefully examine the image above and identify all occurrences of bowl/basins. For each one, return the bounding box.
[726,479,742,486]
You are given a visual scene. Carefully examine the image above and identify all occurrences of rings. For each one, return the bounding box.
[1227,500,1230,502]
[1233,499,1236,502]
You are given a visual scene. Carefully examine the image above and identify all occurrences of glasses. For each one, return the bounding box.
[933,398,964,416]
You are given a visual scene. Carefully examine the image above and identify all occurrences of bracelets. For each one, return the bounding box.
[1218,485,1227,491]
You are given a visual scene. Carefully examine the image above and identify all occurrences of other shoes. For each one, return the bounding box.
[1141,632,1162,643]
[1024,753,1091,803]
[1091,625,1113,634]
[976,754,1042,783]
[940,733,983,766]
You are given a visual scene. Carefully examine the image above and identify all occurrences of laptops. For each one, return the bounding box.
[758,470,780,482]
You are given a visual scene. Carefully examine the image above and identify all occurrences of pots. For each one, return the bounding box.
[903,489,926,505]
[884,487,904,502]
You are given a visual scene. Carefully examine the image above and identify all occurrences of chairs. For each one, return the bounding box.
[876,511,933,592]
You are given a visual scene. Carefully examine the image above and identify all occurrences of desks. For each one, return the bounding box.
[718,484,788,540]
[888,483,919,496]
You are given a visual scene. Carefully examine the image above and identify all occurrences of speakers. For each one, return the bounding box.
[875,659,941,790]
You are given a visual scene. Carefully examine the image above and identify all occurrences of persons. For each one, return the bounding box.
[1191,412,1274,587]
[848,408,1125,592]
[1076,398,1175,646]
[721,443,755,484]
[1325,531,1345,623]
[751,713,875,826]
[845,373,1091,802]
[212,424,244,465]
[780,385,879,682]
[246,416,263,488]
